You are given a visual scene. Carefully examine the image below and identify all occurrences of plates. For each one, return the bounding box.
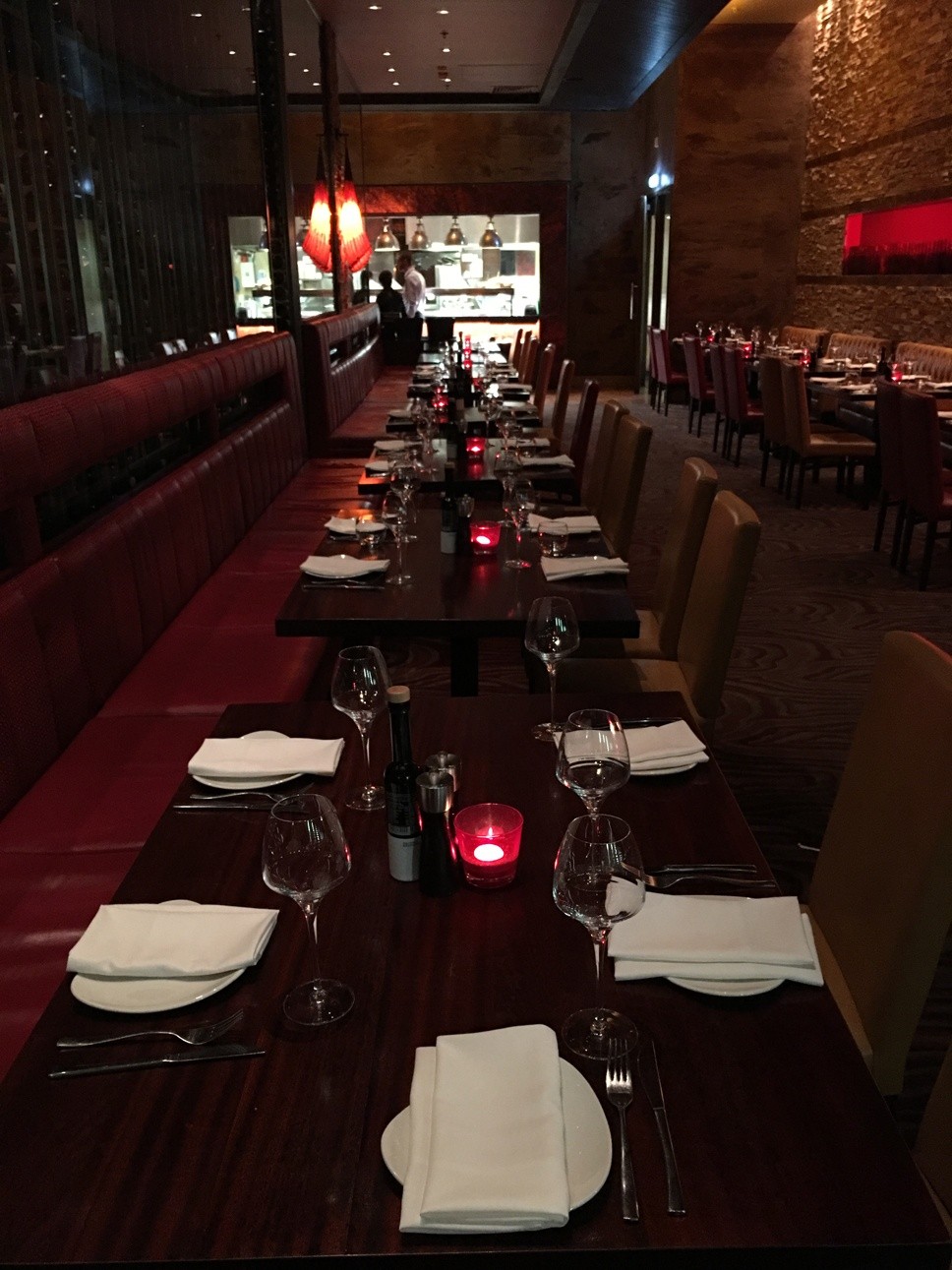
[665,895,785,997]
[70,899,247,1013]
[381,1057,612,1212]
[329,527,355,535]
[193,730,304,790]
[630,762,698,775]
[305,554,370,579]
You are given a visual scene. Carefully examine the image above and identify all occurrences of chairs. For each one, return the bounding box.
[509,326,952,723]
[793,629,952,1154]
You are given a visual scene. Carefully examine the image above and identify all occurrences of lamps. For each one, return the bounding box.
[342,133,373,272]
[374,215,504,251]
[307,132,332,273]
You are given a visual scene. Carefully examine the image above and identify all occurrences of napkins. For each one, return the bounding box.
[555,719,712,771]
[67,906,282,979]
[187,736,347,781]
[606,875,824,992]
[298,439,630,580]
[406,1033,570,1237]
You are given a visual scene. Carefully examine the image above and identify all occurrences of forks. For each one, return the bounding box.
[56,1007,244,1046]
[605,1038,639,1220]
[620,862,777,888]
[190,785,312,802]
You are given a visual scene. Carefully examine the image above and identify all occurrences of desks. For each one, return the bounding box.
[1,695,952,1269]
[276,489,640,693]
[405,364,530,402]
[387,395,543,435]
[801,375,951,495]
[355,436,576,506]
[417,351,510,367]
[835,401,952,499]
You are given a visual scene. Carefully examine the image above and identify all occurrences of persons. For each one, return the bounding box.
[395,249,426,319]
[377,270,408,319]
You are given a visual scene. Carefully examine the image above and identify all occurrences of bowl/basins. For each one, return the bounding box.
[355,512,388,549]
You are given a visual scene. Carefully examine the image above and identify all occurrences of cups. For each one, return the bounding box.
[464,336,472,369]
[454,802,524,888]
[536,521,569,556]
[800,353,811,366]
[466,437,484,458]
[744,343,752,354]
[707,335,715,343]
[470,520,500,555]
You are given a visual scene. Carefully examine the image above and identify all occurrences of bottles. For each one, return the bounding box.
[875,348,892,385]
[447,397,462,459]
[384,686,464,898]
[456,332,464,400]
[439,462,475,556]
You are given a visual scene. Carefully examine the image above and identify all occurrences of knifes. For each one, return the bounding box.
[619,717,683,723]
[173,801,307,810]
[622,864,758,874]
[302,584,386,590]
[637,1036,685,1215]
[48,1046,266,1078]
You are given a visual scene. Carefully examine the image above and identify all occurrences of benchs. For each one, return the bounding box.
[892,340,952,389]
[299,303,416,458]
[0,327,373,1090]
[823,334,891,368]
[773,327,831,363]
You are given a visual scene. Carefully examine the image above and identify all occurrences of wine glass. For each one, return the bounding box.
[524,597,580,742]
[478,371,533,569]
[830,342,841,363]
[552,814,639,1061]
[262,794,357,1026]
[696,320,809,359]
[330,646,392,810]
[555,709,631,892]
[381,341,459,585]
